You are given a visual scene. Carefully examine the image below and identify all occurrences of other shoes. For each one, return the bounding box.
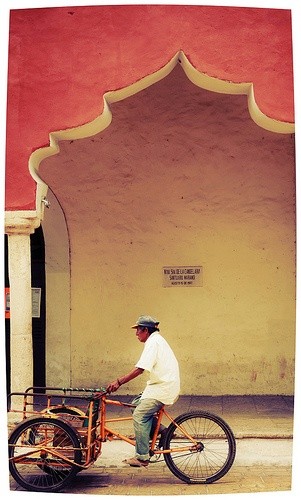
[156,430,175,449]
[122,457,149,466]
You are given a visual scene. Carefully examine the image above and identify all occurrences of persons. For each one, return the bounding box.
[105,316,180,466]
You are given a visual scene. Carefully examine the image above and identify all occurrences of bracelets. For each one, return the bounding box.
[118,378,121,387]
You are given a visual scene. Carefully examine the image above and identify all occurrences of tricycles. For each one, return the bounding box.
[8,387,237,495]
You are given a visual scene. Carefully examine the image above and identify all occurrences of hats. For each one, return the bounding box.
[131,315,160,331]
